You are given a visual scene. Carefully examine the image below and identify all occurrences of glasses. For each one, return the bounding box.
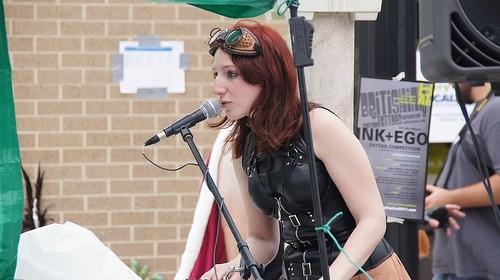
[207,28,263,57]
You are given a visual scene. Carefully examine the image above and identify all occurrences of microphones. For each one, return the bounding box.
[145,99,221,146]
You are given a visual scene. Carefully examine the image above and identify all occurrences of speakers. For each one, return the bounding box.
[419,0,500,84]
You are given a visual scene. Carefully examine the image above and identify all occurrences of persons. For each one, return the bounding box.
[215,121,466,280]
[425,83,500,280]
[199,20,412,280]
